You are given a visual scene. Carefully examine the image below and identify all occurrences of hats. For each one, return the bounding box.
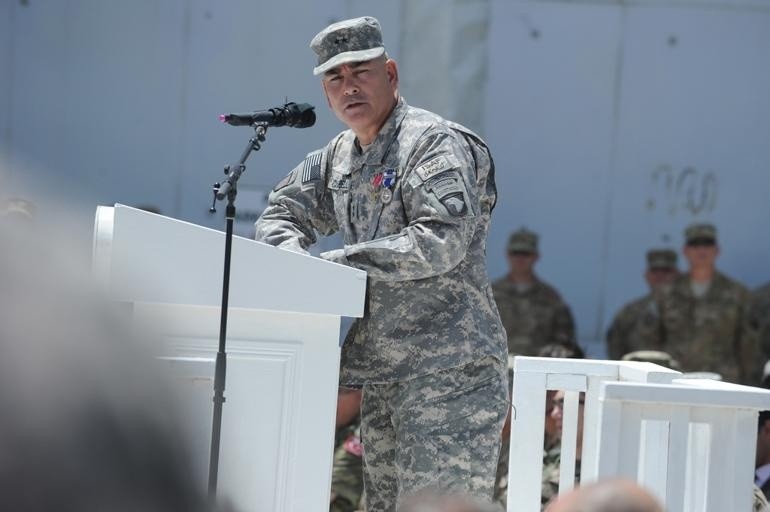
[644,246,679,272]
[310,15,386,76]
[507,230,539,254]
[681,222,720,247]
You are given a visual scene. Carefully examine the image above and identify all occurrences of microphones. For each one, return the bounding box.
[220,101,316,128]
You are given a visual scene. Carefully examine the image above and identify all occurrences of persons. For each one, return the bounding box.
[598,243,685,362]
[654,222,762,384]
[253,16,510,512]
[322,344,770,512]
[485,227,578,360]
